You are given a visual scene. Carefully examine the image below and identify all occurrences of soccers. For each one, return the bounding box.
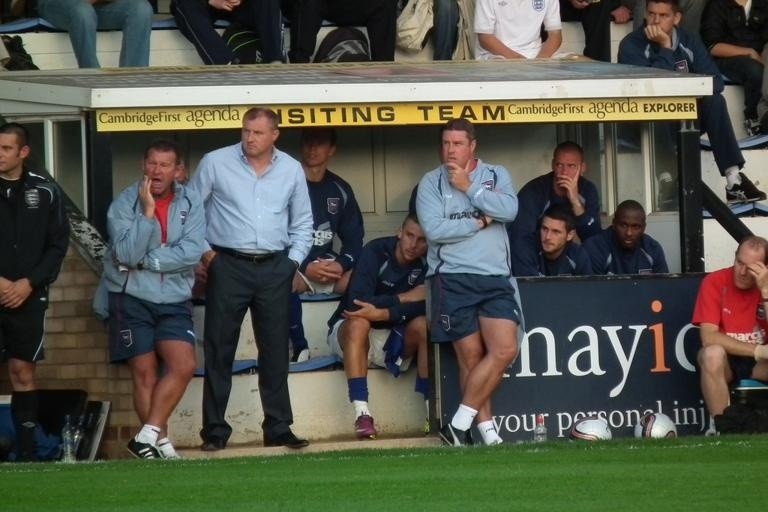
[634,413,677,438]
[568,417,613,440]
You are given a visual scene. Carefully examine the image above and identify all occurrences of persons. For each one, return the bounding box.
[11,0,289,68]
[185,108,313,449]
[414,119,520,444]
[328,213,428,441]
[618,1,767,205]
[585,200,669,273]
[292,128,364,363]
[2,124,69,460]
[692,235,768,435]
[292,0,619,62]
[102,140,206,462]
[506,141,601,277]
[177,155,210,304]
[538,203,593,277]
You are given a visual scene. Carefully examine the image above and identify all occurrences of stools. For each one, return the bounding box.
[729,378,768,408]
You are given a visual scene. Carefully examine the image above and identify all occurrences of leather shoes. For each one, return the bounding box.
[201,440,225,450]
[264,432,308,448]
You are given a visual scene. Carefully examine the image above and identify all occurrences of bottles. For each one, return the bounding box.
[532,414,548,442]
[61,414,80,463]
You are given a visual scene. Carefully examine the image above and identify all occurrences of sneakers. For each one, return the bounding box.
[726,172,765,203]
[126,438,160,460]
[438,423,473,447]
[743,118,761,136]
[158,442,180,460]
[355,416,376,441]
[288,349,308,364]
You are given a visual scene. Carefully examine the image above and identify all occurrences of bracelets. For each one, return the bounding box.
[760,299,768,303]
[138,261,144,270]
[479,215,488,226]
